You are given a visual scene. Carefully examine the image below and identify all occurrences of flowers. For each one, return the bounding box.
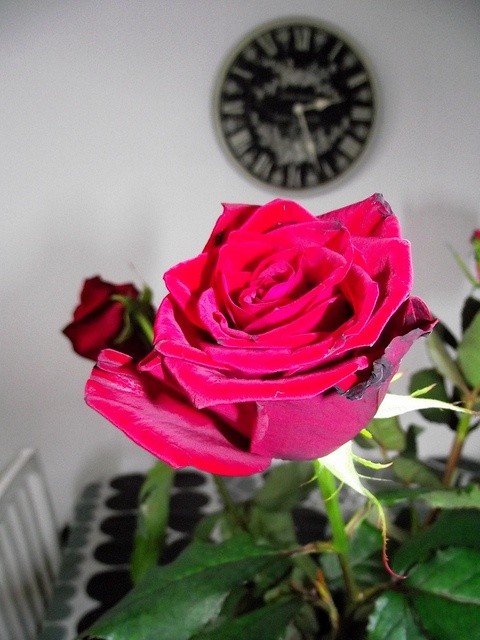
[59,198,480,638]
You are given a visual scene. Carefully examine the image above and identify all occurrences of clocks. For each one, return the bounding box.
[209,14,382,200]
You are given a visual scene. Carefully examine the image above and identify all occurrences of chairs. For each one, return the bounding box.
[0,437,62,640]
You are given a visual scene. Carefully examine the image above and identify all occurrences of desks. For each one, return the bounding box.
[40,457,480,640]
